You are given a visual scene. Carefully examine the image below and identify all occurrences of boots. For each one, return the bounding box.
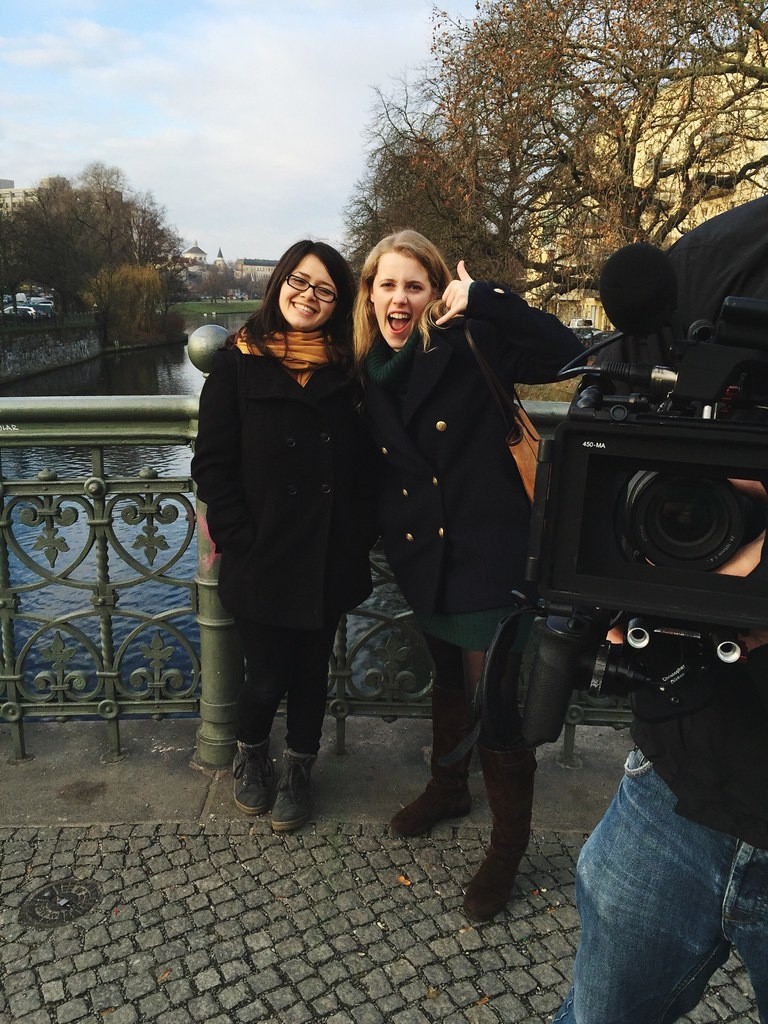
[461,745,537,923]
[390,685,473,837]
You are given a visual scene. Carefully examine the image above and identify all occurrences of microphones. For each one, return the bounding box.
[599,241,679,338]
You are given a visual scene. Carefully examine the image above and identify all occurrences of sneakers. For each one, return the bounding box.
[271,749,318,830]
[232,741,270,815]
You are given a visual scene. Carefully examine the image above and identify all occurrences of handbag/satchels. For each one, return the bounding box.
[464,321,540,503]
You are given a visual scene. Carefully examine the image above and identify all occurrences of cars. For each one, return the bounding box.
[0,306,36,315]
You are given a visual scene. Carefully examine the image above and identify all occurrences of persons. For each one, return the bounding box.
[550,196,768,1023]
[352,230,588,923]
[190,239,373,831]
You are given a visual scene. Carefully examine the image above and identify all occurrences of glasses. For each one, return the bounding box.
[286,273,340,303]
